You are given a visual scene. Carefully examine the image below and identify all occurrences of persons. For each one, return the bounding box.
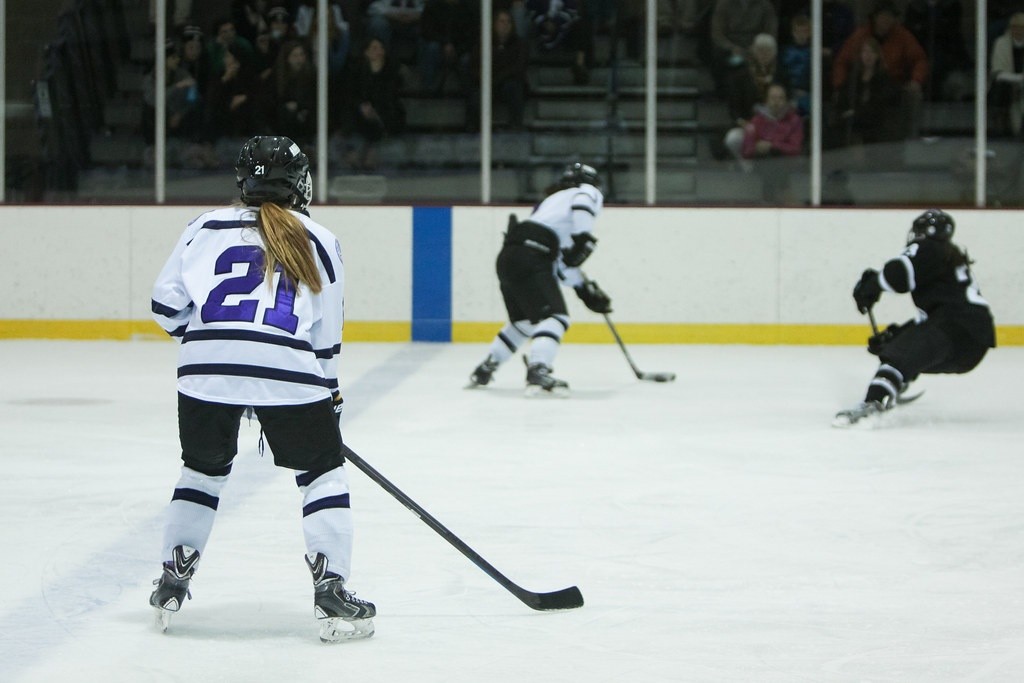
[991,12,1024,137]
[832,208,996,432]
[462,162,614,398]
[148,135,377,644]
[657,0,931,164]
[347,0,646,169]
[139,0,345,134]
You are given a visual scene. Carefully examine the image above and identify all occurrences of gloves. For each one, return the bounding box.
[868,322,902,355]
[852,268,884,314]
[561,231,598,268]
[331,390,344,421]
[573,279,614,315]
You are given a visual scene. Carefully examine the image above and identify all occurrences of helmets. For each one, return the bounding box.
[907,208,956,246]
[236,135,313,211]
[563,161,604,193]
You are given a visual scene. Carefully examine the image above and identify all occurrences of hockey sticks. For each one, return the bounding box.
[867,307,885,364]
[577,265,677,383]
[341,442,584,610]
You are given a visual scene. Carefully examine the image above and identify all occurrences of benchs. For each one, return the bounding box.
[4,3,1022,210]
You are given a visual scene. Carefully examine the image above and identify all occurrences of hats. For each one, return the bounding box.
[181,24,205,43]
[269,7,291,24]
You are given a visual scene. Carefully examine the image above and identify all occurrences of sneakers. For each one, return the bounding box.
[304,551,376,644]
[833,400,892,431]
[463,353,499,389]
[522,354,569,399]
[149,545,201,633]
